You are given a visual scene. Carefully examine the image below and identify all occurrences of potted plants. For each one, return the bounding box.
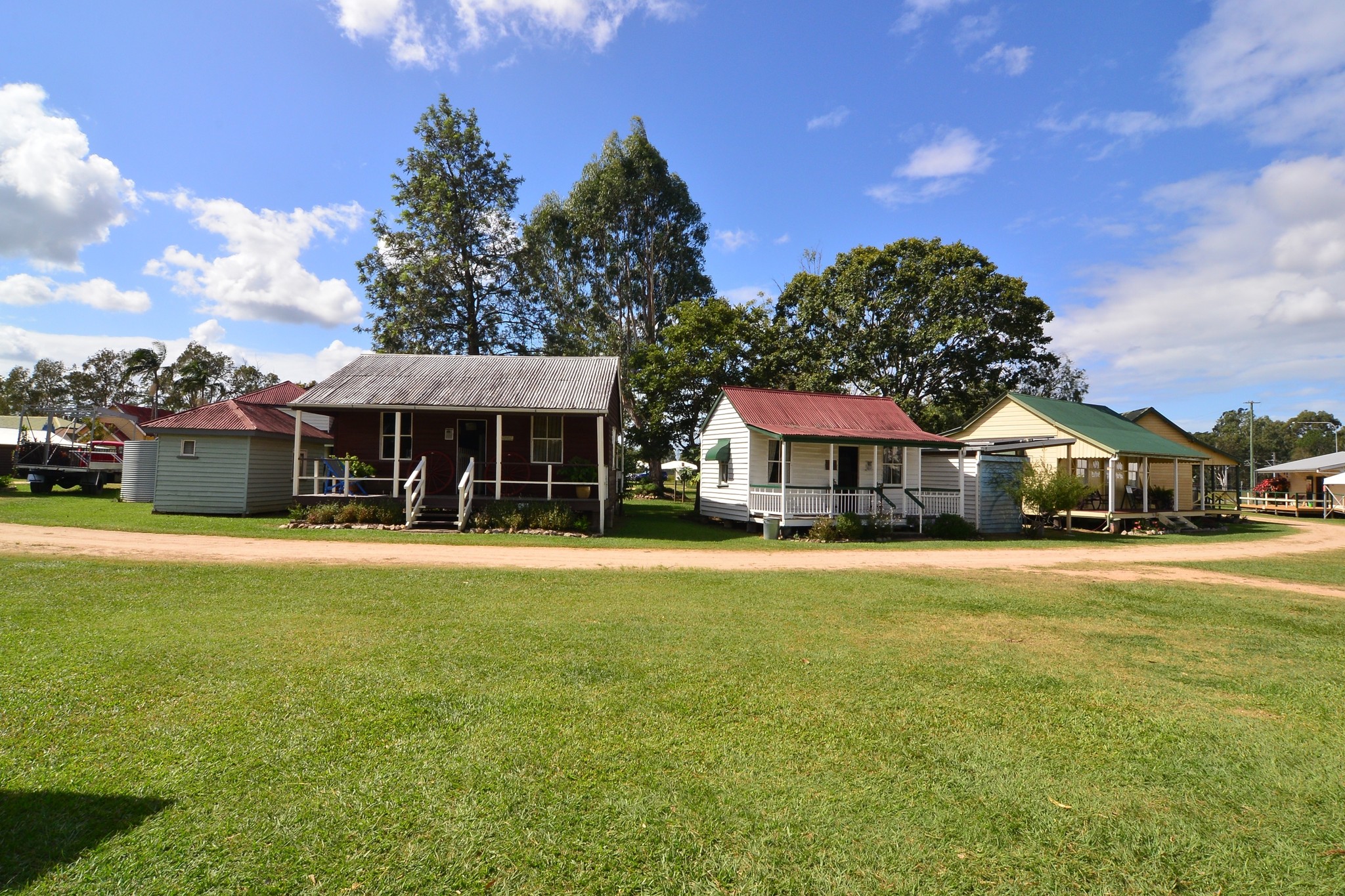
[556,456,598,498]
[1149,485,1175,509]
[329,451,377,493]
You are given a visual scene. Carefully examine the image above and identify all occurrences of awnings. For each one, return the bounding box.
[705,439,731,461]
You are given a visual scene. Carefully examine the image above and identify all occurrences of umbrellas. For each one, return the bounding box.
[635,460,650,472]
[1323,472,1345,485]
[660,461,698,485]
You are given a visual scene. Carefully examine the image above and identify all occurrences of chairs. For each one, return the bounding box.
[321,456,368,496]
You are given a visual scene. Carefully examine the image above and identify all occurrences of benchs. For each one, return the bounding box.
[1290,495,1304,500]
[1079,486,1109,510]
[1131,488,1162,509]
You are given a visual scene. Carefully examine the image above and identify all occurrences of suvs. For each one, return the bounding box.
[634,470,668,483]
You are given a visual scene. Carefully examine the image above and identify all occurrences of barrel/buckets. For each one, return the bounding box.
[763,517,781,540]
[1308,502,1313,507]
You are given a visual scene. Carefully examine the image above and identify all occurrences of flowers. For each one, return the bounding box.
[1251,476,1291,499]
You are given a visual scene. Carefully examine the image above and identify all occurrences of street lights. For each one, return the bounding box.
[1292,421,1338,453]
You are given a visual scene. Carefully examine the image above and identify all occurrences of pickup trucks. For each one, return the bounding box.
[12,440,124,496]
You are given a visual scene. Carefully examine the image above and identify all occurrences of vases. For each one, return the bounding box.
[1266,493,1275,502]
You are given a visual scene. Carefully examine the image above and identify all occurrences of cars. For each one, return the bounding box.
[626,473,638,481]
